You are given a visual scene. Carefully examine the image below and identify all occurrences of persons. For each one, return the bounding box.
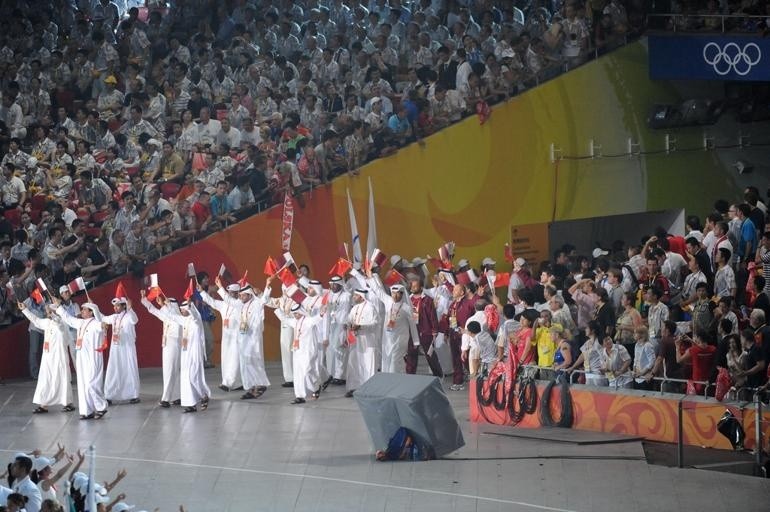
[0,0,769,416]
[0,443,129,511]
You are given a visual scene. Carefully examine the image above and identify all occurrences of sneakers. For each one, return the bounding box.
[449,383,466,390]
[438,376,447,384]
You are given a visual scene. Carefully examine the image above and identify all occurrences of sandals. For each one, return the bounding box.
[310,385,321,401]
[281,382,294,387]
[344,390,355,397]
[107,400,113,406]
[255,386,267,399]
[79,412,95,420]
[200,396,209,411]
[290,398,307,404]
[32,405,48,414]
[162,401,170,407]
[130,397,141,404]
[182,405,197,413]
[218,385,229,392]
[333,377,346,385]
[240,392,255,399]
[322,375,333,391]
[62,405,76,412]
[94,408,108,420]
[173,398,181,405]
[235,385,243,391]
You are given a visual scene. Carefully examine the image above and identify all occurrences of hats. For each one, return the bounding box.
[111,502,135,512]
[103,75,118,85]
[370,97,385,105]
[25,156,38,168]
[145,138,160,146]
[458,259,469,268]
[84,492,110,510]
[70,472,87,482]
[80,483,104,496]
[12,452,34,463]
[390,255,402,268]
[73,476,90,491]
[32,456,55,472]
[592,248,609,259]
[516,257,526,267]
[482,257,497,266]
[412,257,428,267]
